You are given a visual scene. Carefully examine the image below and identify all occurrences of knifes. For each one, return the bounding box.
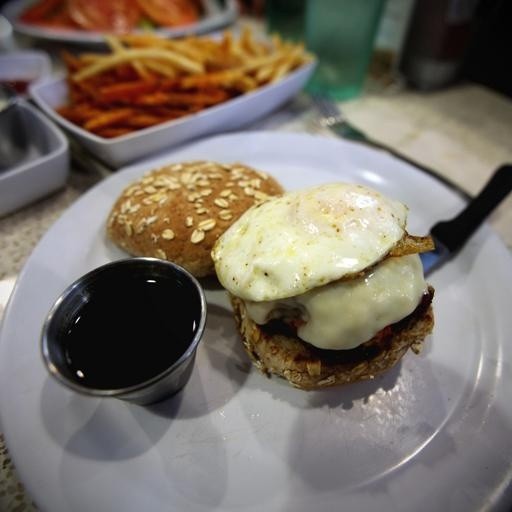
[418,164,512,275]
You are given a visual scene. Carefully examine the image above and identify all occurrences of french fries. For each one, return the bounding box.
[48,27,313,137]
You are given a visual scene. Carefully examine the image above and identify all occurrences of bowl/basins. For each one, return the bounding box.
[38,256,210,407]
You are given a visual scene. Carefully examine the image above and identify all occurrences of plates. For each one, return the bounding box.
[29,24,319,171]
[0,96,75,221]
[4,0,240,49]
[0,45,56,115]
[0,131,512,511]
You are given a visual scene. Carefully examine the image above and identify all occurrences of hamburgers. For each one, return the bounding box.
[104,157,438,393]
